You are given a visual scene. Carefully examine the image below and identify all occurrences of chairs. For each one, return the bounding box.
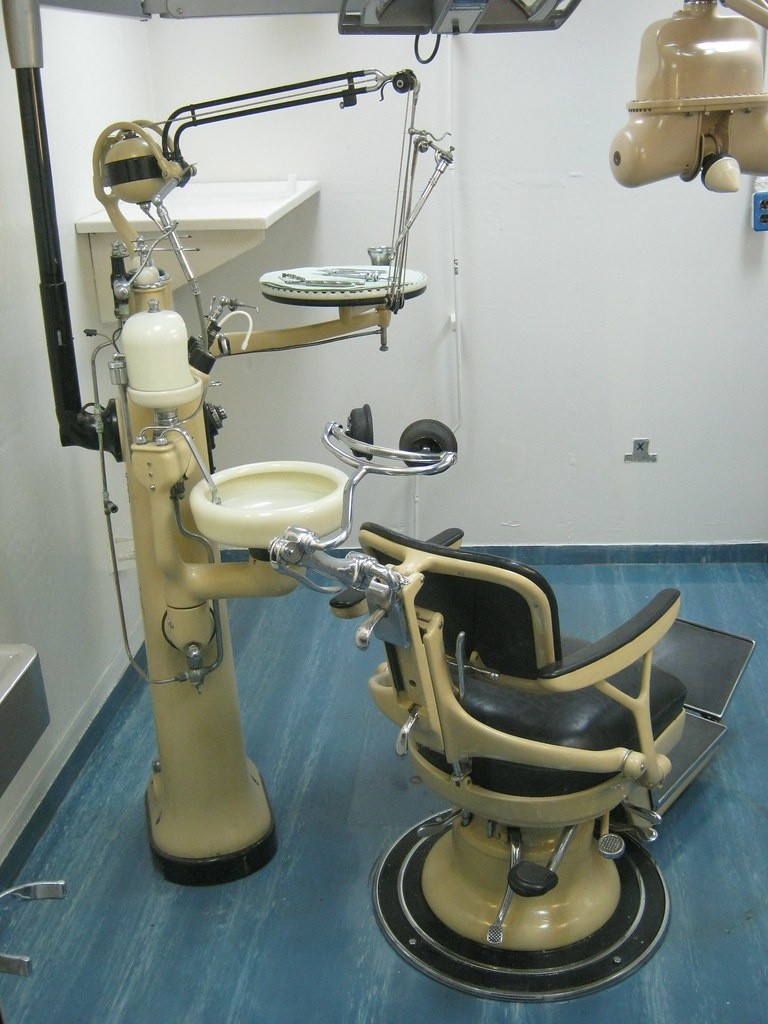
[329,524,755,1001]
